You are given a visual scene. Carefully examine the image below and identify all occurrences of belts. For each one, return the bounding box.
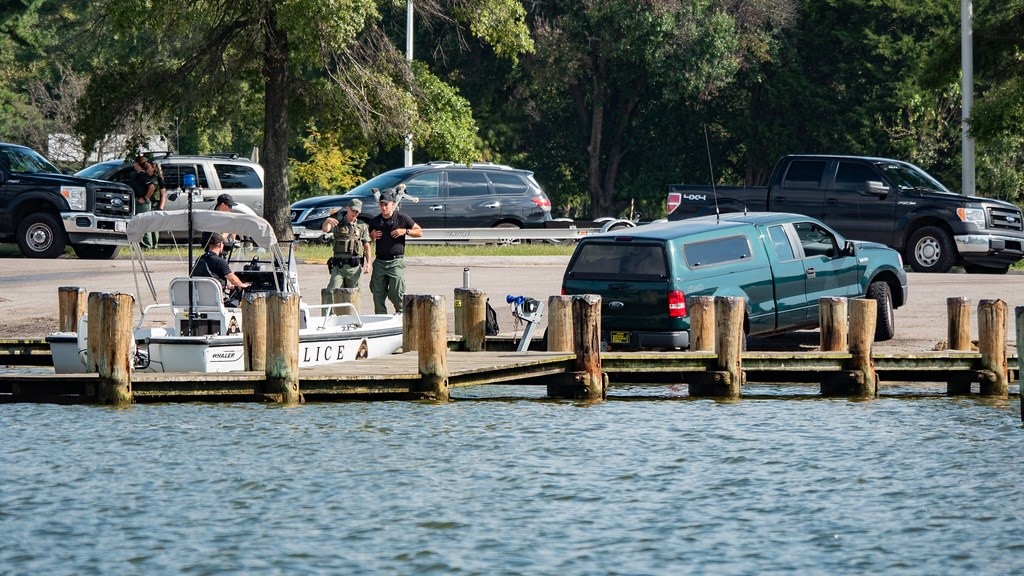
[376,255,403,260]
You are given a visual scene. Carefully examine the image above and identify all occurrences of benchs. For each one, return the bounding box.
[169,276,242,336]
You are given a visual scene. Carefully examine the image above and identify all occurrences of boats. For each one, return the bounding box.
[41,209,406,376]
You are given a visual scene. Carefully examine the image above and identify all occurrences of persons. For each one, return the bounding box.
[200,193,239,260]
[368,193,423,315]
[321,198,372,316]
[188,231,253,307]
[129,156,166,251]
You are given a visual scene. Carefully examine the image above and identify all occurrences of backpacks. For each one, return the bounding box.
[486,298,499,336]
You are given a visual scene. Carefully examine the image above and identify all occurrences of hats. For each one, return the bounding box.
[209,234,224,245]
[348,198,363,213]
[379,193,393,202]
[217,194,238,206]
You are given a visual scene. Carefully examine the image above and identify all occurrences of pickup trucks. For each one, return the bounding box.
[666,152,1024,274]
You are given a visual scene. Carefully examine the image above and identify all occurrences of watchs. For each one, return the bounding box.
[406,228,410,235]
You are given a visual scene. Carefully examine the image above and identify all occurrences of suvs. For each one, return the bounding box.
[0,142,136,260]
[288,160,552,247]
[561,210,908,356]
[71,150,267,245]
